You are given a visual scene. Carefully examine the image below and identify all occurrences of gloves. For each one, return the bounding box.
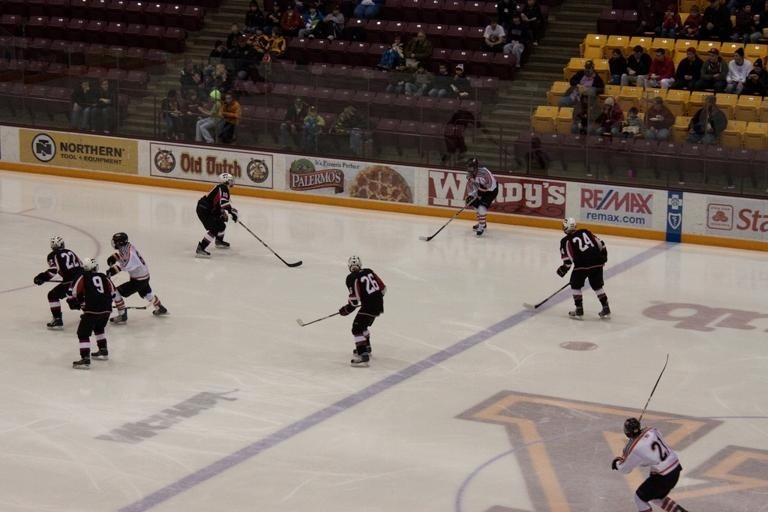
[557,264,570,276]
[34,273,49,285]
[77,295,84,304]
[339,306,349,316]
[106,256,121,276]
[466,190,483,206]
[600,248,607,265]
[221,208,238,223]
[612,457,623,470]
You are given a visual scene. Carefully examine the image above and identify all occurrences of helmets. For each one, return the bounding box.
[210,90,221,99]
[465,158,478,172]
[562,217,576,234]
[218,172,233,185]
[51,235,64,249]
[624,417,641,438]
[348,256,362,272]
[111,233,128,249]
[654,97,662,104]
[83,257,99,272]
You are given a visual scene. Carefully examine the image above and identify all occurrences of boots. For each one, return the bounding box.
[196,235,230,256]
[473,222,486,236]
[569,303,583,316]
[153,304,166,315]
[47,312,63,327]
[73,344,108,364]
[599,302,610,316]
[110,308,127,322]
[351,337,371,363]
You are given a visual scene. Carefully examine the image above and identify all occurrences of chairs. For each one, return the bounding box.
[513,1,768,193]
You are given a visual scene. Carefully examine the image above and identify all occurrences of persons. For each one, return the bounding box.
[160,59,244,148]
[465,158,498,235]
[379,30,475,102]
[558,1,767,148]
[195,173,239,258]
[557,217,611,318]
[277,95,375,158]
[483,1,543,66]
[207,0,377,89]
[34,232,167,370]
[340,255,388,367]
[612,417,688,512]
[67,77,115,136]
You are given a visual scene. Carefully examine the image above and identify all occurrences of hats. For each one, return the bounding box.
[294,97,318,112]
[736,48,744,56]
[753,58,762,68]
[584,60,593,67]
[605,97,614,106]
[687,47,718,55]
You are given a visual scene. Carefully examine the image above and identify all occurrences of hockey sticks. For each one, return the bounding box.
[80,297,155,309]
[296,305,362,327]
[622,350,670,456]
[419,196,476,241]
[237,220,302,267]
[522,284,572,310]
